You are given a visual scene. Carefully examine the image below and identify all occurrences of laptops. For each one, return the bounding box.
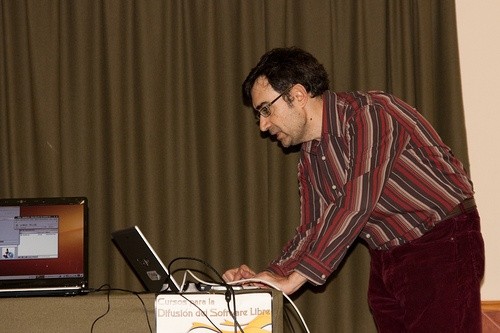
[0,197,89,299]
[111,225,261,294]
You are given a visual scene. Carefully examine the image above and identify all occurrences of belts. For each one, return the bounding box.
[440,198,476,220]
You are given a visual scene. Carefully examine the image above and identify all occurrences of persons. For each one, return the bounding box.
[221,46,486,333]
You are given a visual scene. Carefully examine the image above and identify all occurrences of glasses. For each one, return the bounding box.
[255,86,292,119]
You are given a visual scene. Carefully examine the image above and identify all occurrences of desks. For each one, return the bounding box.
[0,290,283,333]
